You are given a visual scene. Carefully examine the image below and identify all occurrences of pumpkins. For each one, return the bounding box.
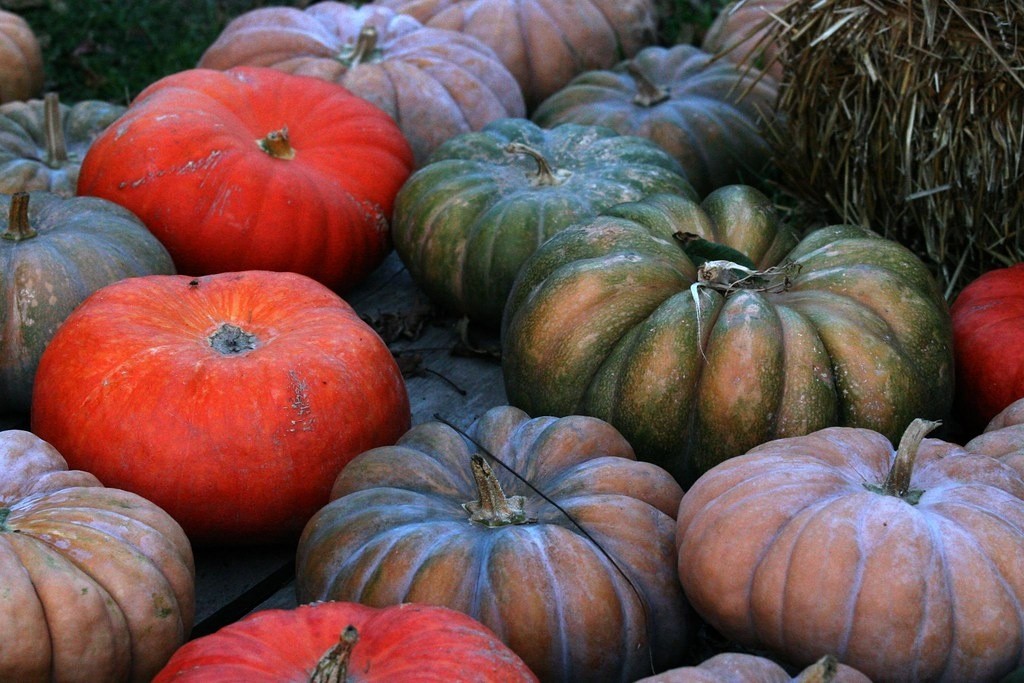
[0,0,1024,683]
